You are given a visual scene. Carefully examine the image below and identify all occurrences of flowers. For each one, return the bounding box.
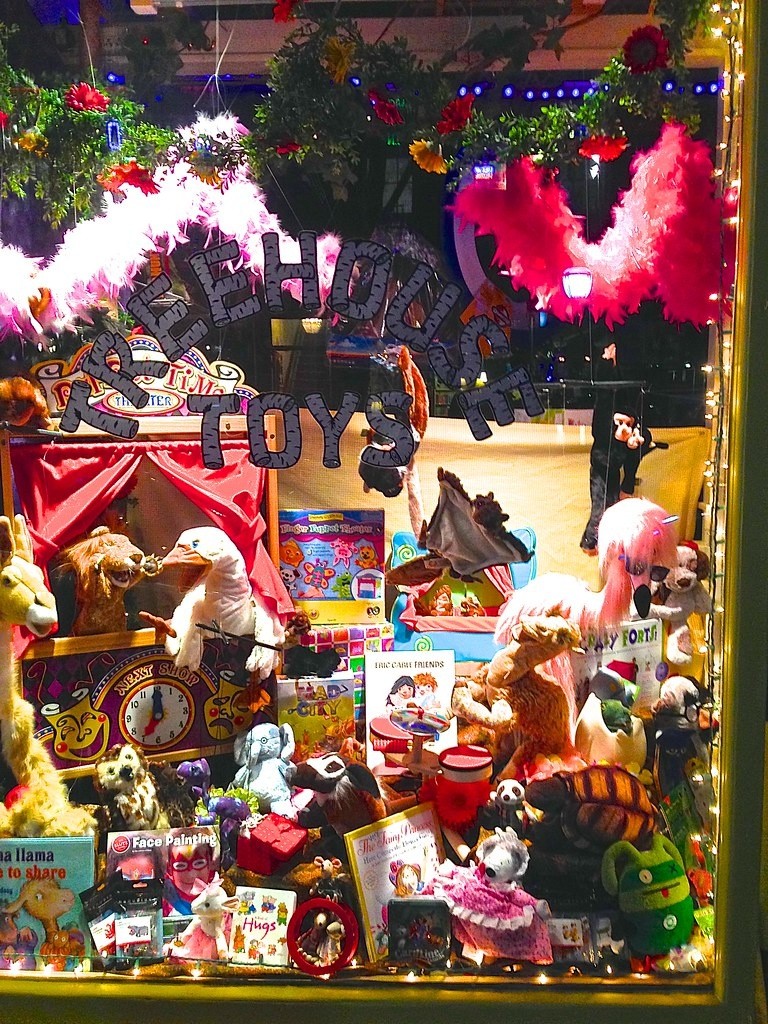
[319,36,357,86]
[622,24,672,75]
[275,133,301,155]
[273,0,311,23]
[16,127,48,158]
[366,89,405,126]
[436,92,475,134]
[187,149,227,186]
[577,136,629,162]
[409,138,448,175]
[96,160,162,204]
[63,81,110,114]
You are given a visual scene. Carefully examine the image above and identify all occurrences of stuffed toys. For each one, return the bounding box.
[0,344,717,980]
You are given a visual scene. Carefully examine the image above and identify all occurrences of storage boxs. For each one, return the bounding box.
[275,507,400,766]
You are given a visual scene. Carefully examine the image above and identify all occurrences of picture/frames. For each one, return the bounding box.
[344,799,446,962]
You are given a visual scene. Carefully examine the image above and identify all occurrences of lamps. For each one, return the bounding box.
[562,266,593,312]
[301,317,323,335]
